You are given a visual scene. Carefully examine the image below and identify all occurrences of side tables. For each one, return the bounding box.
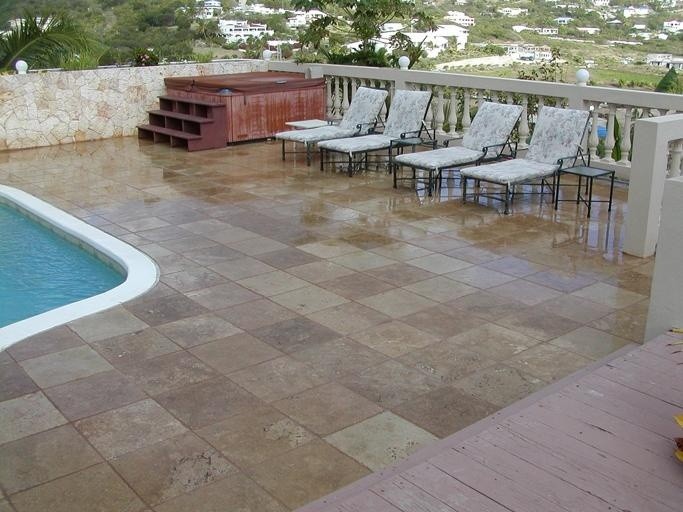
[554,165,615,218]
